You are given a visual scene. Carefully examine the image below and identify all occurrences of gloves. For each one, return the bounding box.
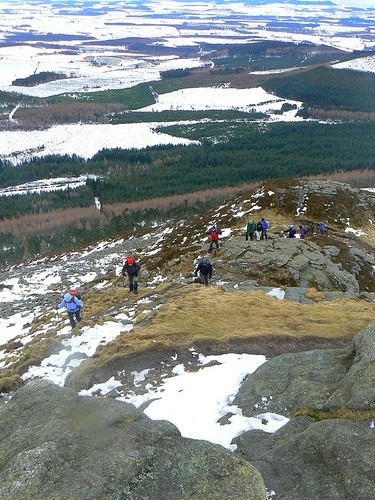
[56,307,59,309]
[77,305,81,308]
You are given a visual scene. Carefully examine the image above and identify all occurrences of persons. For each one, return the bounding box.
[122,258,141,294]
[246,218,268,240]
[320,222,326,234]
[284,225,296,238]
[310,223,316,234]
[195,260,213,285]
[55,293,83,328]
[207,227,222,251]
[299,226,305,238]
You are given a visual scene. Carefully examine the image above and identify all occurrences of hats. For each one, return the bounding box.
[128,258,133,264]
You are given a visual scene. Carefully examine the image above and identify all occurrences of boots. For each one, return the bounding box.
[128,284,133,292]
[134,286,138,294]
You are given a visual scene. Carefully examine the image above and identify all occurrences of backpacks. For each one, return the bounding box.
[65,290,81,304]
